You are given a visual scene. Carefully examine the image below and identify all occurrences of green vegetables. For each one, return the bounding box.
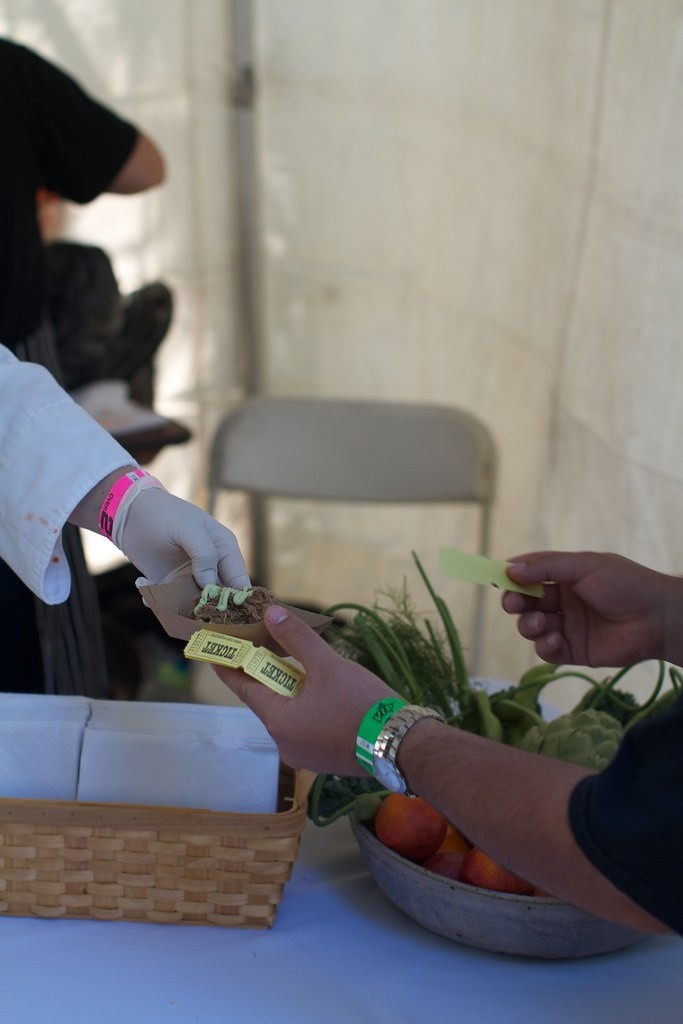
[302,551,683,827]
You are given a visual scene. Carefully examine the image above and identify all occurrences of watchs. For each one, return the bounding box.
[372,704,447,799]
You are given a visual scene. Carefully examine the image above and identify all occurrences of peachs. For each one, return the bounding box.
[374,794,548,897]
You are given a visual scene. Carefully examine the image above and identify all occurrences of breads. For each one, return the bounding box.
[188,584,275,625]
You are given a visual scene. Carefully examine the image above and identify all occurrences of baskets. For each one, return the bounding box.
[0,768,319,931]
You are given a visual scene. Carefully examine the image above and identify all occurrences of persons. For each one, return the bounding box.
[0,37,165,696]
[210,551,683,940]
[0,342,251,612]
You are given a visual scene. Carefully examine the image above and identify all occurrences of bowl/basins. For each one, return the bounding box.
[348,815,650,957]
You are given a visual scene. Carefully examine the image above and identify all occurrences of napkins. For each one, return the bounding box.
[0,692,278,820]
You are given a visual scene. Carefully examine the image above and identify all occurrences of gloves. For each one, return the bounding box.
[111,476,252,606]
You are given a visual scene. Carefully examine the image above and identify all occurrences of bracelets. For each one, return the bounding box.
[354,697,407,779]
[99,469,164,542]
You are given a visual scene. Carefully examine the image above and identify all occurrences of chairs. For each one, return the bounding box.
[199,393,498,678]
